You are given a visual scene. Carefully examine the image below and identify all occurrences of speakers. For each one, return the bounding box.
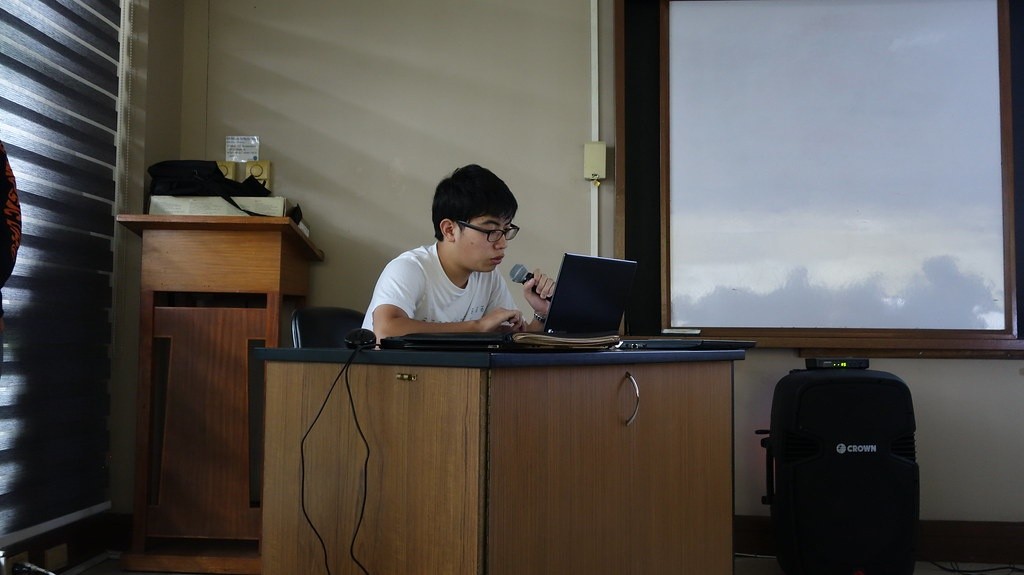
[769,369,920,575]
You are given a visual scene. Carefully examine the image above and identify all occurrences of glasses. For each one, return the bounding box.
[456,220,521,243]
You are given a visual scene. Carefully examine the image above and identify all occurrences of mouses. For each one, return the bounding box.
[345,328,376,350]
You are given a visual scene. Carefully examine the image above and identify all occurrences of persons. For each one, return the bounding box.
[360,164,557,351]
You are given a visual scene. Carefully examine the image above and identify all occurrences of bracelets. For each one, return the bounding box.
[534,312,546,323]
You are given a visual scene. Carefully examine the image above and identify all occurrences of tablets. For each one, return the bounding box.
[614,339,757,353]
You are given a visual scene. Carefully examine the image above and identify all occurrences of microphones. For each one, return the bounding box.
[509,264,553,304]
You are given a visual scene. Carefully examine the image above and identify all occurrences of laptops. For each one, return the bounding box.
[405,333,525,354]
[528,253,639,349]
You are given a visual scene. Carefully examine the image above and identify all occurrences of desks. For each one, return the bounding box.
[251,340,756,575]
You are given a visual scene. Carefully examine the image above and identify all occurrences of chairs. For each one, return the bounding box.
[292,307,368,348]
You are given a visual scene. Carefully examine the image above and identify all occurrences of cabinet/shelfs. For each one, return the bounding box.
[117,214,325,554]
